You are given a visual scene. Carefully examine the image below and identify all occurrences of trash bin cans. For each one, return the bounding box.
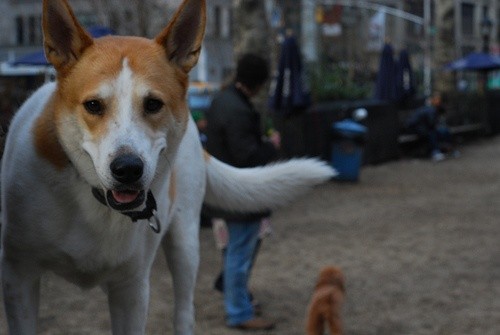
[324,120,367,183]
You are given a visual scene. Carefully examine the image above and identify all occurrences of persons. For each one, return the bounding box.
[197,53,280,329]
[403,96,462,163]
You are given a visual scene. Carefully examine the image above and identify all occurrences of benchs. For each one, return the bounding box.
[397,109,485,157]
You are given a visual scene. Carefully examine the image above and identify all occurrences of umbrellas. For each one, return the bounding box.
[447,52,500,70]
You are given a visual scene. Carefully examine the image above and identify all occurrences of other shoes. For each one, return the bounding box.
[228,317,275,330]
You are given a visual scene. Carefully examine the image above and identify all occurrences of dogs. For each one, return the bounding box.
[1,0,342,335]
[305,265,347,335]
